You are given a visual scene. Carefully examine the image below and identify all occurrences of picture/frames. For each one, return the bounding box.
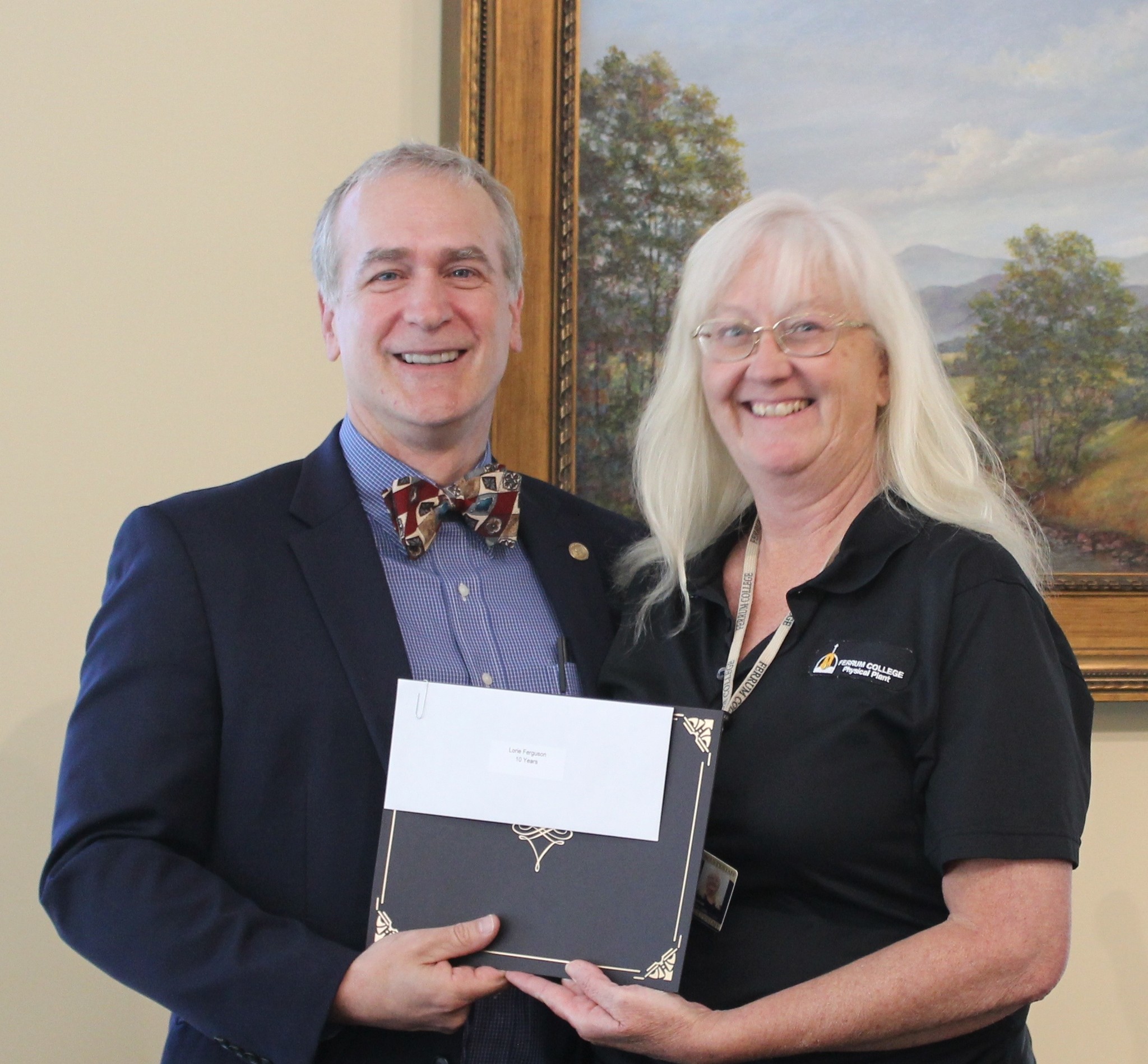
[455,1,1147,702]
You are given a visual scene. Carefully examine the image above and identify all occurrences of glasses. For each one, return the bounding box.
[690,312,878,363]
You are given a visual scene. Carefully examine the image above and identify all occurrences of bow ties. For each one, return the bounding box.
[381,469,523,560]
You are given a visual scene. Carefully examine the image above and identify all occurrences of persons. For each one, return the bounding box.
[506,192,1092,1064]
[34,141,661,1064]
